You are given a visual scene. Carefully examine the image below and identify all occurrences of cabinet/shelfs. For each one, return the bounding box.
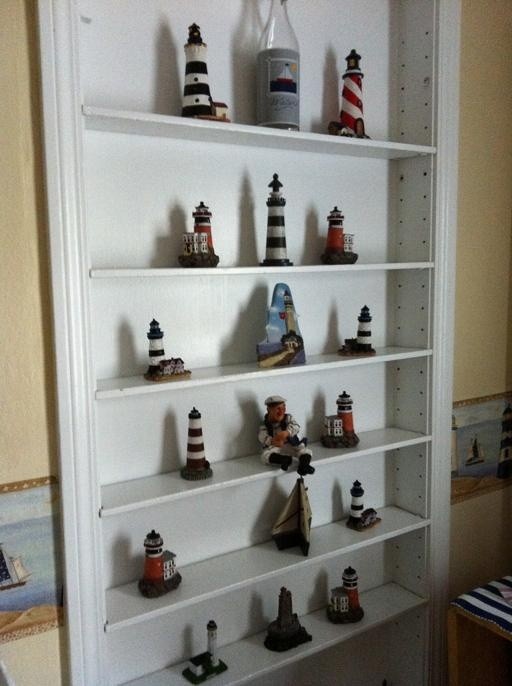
[82,104,437,686]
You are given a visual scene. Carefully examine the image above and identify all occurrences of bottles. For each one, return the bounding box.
[253,0,303,132]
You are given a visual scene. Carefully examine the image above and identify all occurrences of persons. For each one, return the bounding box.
[256,396,314,474]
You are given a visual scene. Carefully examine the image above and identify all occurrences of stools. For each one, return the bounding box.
[448,575,512,686]
